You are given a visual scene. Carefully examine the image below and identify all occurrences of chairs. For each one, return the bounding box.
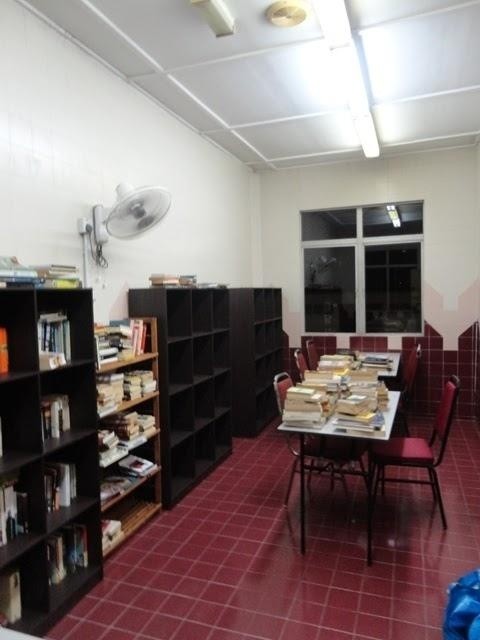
[271,338,462,531]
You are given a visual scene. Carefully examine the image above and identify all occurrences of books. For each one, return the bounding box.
[281,348,394,437]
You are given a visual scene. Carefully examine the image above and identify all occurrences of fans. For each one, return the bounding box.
[81,178,173,244]
[309,254,337,275]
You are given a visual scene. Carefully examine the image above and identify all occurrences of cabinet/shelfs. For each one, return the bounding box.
[2,288,105,636]
[129,288,281,510]
[305,287,342,332]
[92,316,163,560]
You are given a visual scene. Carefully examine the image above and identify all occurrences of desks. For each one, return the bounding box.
[275,388,402,566]
